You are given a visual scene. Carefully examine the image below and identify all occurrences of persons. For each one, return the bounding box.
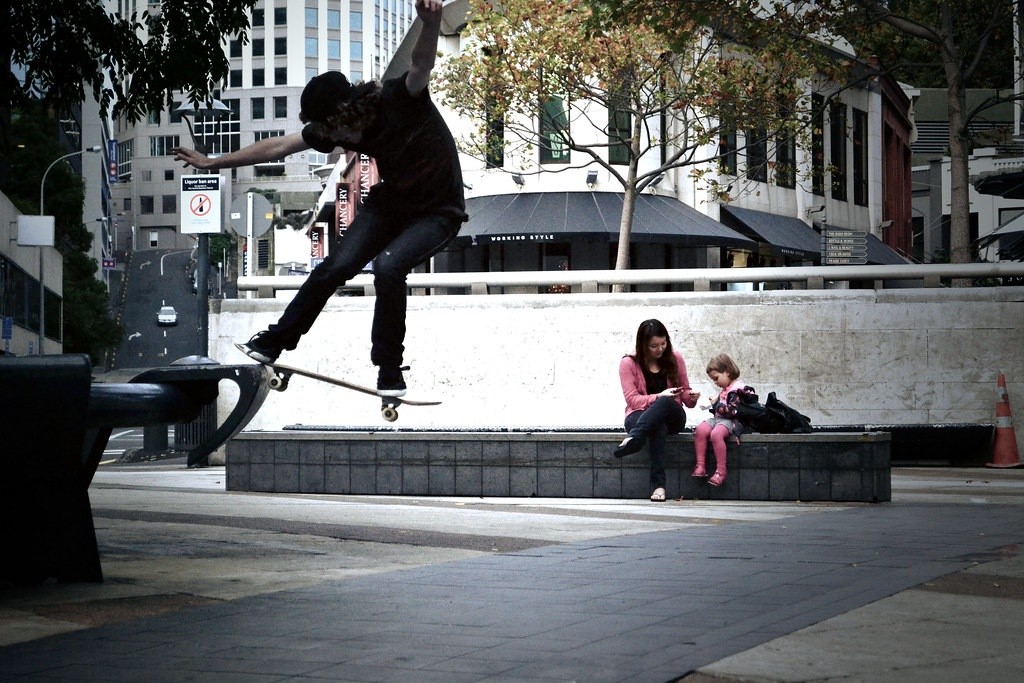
[692,356,749,486]
[171,0,469,397]
[613,318,701,502]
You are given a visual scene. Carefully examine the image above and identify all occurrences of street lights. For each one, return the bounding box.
[170,99,235,377]
[37,143,103,356]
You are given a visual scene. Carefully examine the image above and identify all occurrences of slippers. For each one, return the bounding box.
[650,492,666,501]
[614,437,643,458]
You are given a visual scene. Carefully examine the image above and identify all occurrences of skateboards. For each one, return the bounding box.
[232,340,442,423]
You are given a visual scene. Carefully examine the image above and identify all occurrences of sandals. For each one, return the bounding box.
[691,464,708,477]
[708,469,727,487]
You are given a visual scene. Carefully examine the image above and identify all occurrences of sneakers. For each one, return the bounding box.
[234,330,282,364]
[377,363,410,397]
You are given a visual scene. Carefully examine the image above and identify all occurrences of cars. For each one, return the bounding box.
[157,306,178,325]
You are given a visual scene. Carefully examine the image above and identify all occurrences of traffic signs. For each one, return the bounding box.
[823,229,869,265]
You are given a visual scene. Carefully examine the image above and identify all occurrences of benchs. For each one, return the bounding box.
[225,430,891,505]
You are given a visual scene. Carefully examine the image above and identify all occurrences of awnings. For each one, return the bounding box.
[813,222,910,265]
[456,191,759,250]
[720,203,821,258]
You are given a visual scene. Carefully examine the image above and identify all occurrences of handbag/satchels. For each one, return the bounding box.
[765,391,813,433]
[737,389,789,434]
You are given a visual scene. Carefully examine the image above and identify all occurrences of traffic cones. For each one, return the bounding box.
[983,372,1024,469]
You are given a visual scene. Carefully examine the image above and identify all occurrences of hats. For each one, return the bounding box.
[300,71,374,119]
